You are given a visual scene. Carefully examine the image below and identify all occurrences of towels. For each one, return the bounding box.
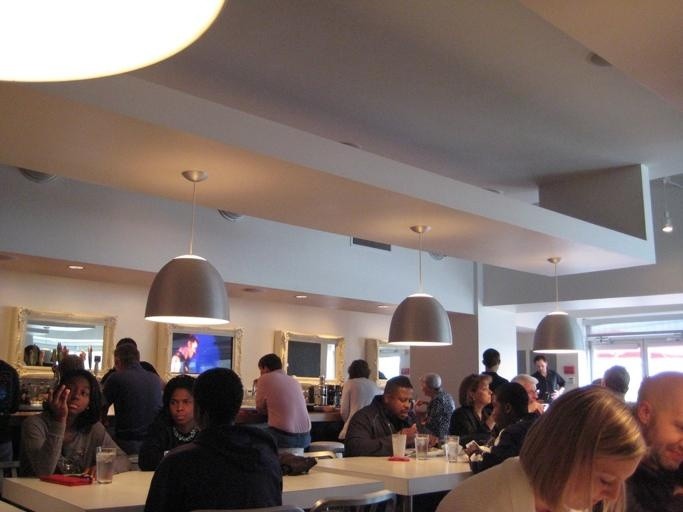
[40,474,91,487]
[388,456,410,462]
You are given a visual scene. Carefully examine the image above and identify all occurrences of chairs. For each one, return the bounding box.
[286,450,337,461]
[310,489,399,512]
[188,503,305,512]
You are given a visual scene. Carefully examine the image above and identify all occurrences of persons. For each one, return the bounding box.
[170,334,200,373]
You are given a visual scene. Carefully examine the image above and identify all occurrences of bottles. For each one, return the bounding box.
[326,383,342,409]
[17,380,51,405]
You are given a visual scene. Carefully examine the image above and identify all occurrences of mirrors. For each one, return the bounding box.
[365,338,412,388]
[10,305,118,381]
[273,329,347,386]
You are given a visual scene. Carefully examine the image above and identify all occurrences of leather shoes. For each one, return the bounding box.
[268,426,308,437]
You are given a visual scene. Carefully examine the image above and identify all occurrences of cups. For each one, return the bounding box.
[391,433,406,457]
[443,434,459,463]
[414,433,429,460]
[95,445,116,484]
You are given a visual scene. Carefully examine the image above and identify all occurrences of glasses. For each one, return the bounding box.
[528,388,540,394]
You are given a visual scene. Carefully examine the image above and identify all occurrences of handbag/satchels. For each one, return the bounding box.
[277,451,318,476]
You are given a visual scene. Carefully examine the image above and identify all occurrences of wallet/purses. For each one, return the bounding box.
[38,473,92,486]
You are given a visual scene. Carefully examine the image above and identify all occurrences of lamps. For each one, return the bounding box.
[386,225,454,347]
[660,175,675,235]
[532,255,585,354]
[143,169,231,328]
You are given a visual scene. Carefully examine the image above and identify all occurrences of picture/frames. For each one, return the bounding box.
[154,321,246,386]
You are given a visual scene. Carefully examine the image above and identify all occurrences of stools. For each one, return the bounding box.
[278,447,305,458]
[308,441,346,459]
